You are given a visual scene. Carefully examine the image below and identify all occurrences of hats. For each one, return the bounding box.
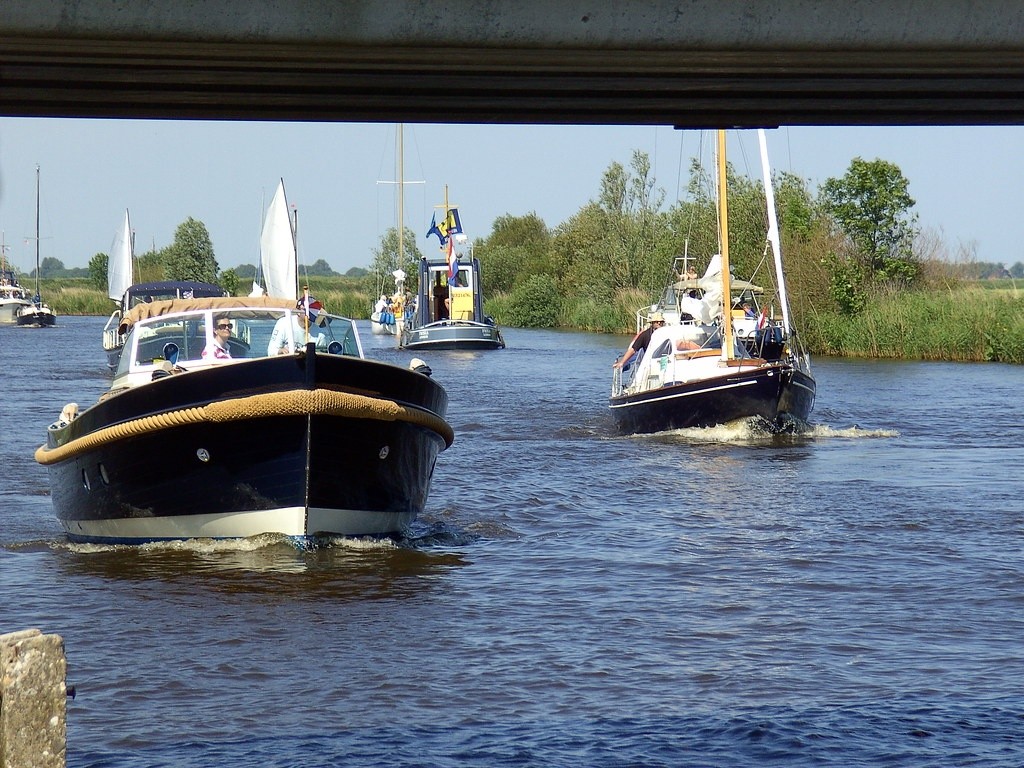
[647,309,663,322]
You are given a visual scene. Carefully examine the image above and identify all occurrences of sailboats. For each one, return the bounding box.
[371,124,414,336]
[16,169,56,327]
[108,208,135,326]
[247,178,300,300]
[610,129,816,437]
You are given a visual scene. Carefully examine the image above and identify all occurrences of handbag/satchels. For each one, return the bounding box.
[103,310,126,349]
[385,313,390,325]
[390,313,394,324]
[380,312,385,325]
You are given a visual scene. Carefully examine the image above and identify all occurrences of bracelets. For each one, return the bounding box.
[619,362,623,366]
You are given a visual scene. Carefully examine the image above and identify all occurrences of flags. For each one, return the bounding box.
[755,306,767,340]
[426,208,463,245]
[444,236,460,288]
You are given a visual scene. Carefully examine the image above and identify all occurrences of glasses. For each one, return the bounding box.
[215,324,233,329]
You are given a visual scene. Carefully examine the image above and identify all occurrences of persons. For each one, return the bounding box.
[202,314,234,359]
[615,312,672,370]
[2,291,20,299]
[375,287,413,314]
[443,277,464,311]
[267,296,330,357]
[144,295,152,303]
[742,303,755,320]
[672,264,698,324]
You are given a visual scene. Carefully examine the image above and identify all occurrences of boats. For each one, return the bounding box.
[102,281,250,372]
[399,184,505,350]
[0,231,33,323]
[34,296,454,545]
[635,240,787,359]
